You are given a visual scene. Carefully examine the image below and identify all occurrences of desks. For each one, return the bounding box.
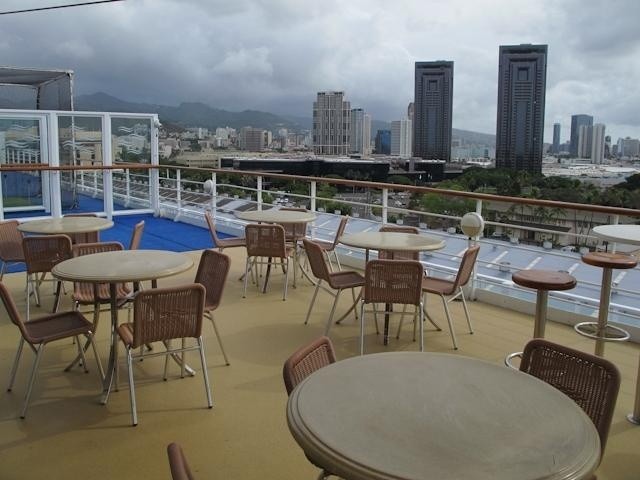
[17,216,114,304]
[337,232,447,339]
[285,351,601,479]
[593,224,640,244]
[236,210,318,287]
[51,249,196,405]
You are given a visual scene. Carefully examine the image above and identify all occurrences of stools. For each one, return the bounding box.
[574,252,638,357]
[504,270,577,371]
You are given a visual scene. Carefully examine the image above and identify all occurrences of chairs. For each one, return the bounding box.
[1,219,26,285]
[279,208,309,272]
[414,245,480,351]
[303,239,380,339]
[69,241,132,364]
[180,250,232,379]
[204,211,255,284]
[62,214,99,244]
[299,217,348,277]
[130,220,145,249]
[167,443,196,480]
[115,283,213,426]
[1,283,107,420]
[360,258,424,351]
[378,226,420,261]
[21,234,73,306]
[283,337,336,399]
[242,224,296,302]
[520,339,622,476]
